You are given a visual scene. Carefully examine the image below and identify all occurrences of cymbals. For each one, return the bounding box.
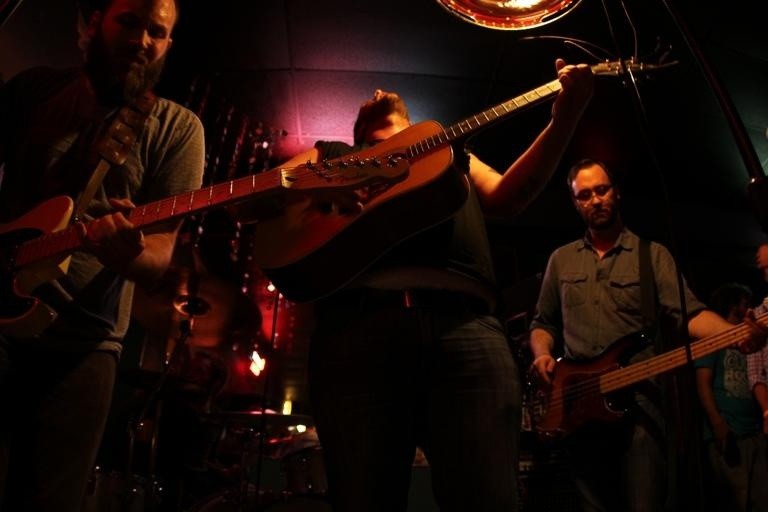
[131,268,261,356]
[120,368,162,388]
[205,407,309,428]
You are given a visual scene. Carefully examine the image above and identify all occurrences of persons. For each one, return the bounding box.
[239,59,596,511]
[0,2,205,510]
[745,243,767,434]
[691,285,766,510]
[531,156,767,507]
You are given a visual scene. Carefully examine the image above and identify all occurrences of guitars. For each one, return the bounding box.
[1,156,409,340]
[251,56,648,305]
[522,299,767,438]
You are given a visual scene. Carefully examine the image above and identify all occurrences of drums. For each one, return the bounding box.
[101,471,161,510]
[192,488,345,510]
